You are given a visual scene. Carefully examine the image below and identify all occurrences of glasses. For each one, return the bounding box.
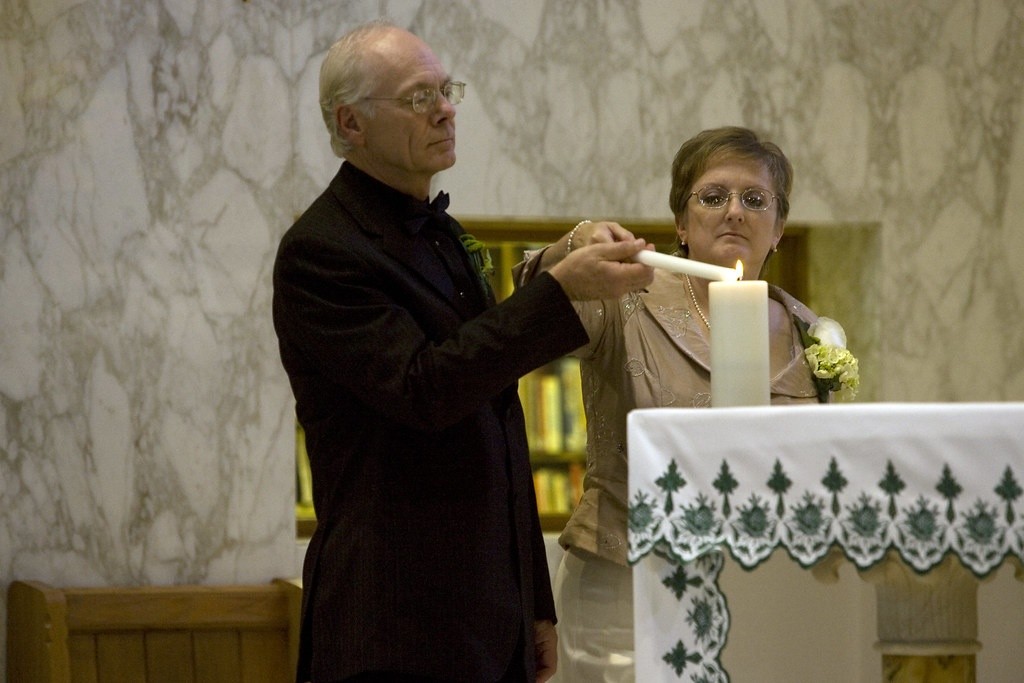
[681,185,778,214]
[363,81,466,113]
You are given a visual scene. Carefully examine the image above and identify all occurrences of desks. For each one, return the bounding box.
[625,399,1024,683]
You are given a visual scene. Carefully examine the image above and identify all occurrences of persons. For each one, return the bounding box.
[511,126,860,683]
[272,25,655,683]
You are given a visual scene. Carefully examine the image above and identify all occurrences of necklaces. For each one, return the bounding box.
[686,274,711,331]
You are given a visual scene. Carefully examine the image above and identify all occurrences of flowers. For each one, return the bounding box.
[793,314,862,403]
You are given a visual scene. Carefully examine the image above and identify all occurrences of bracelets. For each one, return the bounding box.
[567,219,592,255]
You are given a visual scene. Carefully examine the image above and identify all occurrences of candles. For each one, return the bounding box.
[708,260,772,407]
[635,250,738,282]
